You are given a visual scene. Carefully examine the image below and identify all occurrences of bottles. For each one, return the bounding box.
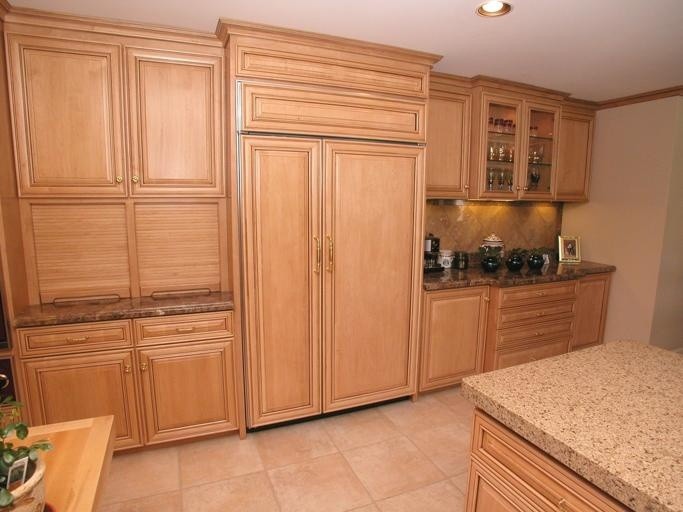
[455,249,469,270]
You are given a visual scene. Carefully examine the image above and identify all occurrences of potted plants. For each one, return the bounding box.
[1,374,53,512]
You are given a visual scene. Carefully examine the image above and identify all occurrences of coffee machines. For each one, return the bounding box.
[424,232,444,273]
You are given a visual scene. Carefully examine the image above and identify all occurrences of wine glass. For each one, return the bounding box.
[532,168,540,192]
[505,168,514,190]
[497,170,505,191]
[487,168,495,192]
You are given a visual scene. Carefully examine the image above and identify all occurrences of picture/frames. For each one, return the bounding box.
[559,236,581,263]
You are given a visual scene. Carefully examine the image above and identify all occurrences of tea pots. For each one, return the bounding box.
[480,234,505,263]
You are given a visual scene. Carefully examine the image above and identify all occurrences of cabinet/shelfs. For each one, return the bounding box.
[214,17,443,431]
[484,271,580,370]
[572,272,612,351]
[555,96,594,201]
[469,74,562,202]
[425,71,469,200]
[8,309,247,450]
[4,6,226,199]
[419,285,490,391]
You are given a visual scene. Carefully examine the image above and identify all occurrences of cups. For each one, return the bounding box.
[529,126,539,139]
[438,249,453,268]
[489,143,515,162]
[529,146,543,163]
[488,117,514,135]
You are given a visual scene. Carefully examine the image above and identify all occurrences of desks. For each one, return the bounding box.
[1,416,117,512]
